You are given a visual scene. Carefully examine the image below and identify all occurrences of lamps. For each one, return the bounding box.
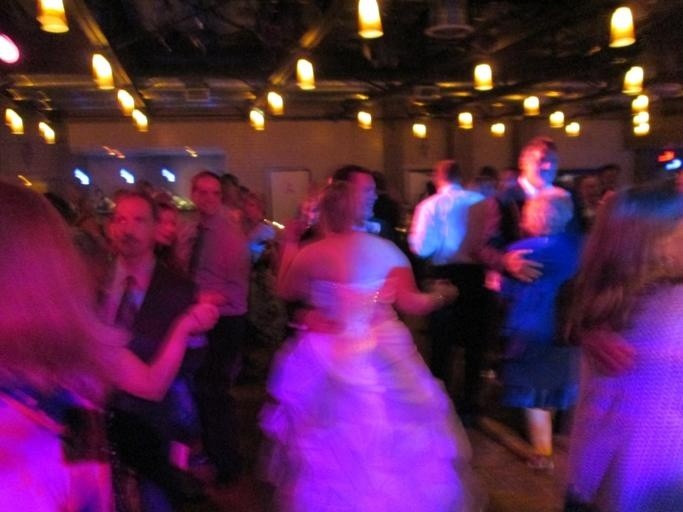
[296,59,315,90]
[474,63,493,91]
[458,112,474,129]
[609,5,637,48]
[358,2,383,38]
[621,65,644,94]
[524,95,539,116]
[550,110,565,128]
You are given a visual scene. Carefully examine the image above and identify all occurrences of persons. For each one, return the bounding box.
[476,137,561,286]
[562,173,683,511]
[32,158,620,511]
[0,178,113,512]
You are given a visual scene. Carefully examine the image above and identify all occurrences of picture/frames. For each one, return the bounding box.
[264,165,313,223]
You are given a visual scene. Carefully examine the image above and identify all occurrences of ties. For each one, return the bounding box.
[188,223,206,278]
[113,278,139,329]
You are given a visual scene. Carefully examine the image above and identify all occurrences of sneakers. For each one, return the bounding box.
[530,456,557,475]
